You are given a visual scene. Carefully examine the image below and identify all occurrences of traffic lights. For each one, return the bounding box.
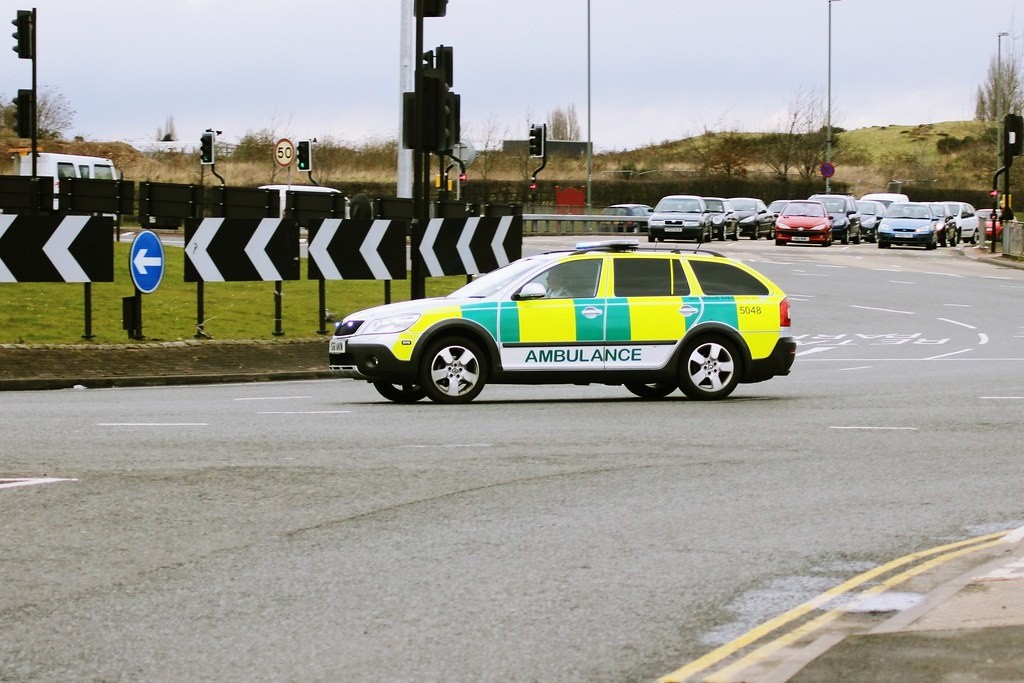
[12,89,32,140]
[10,8,35,62]
[990,190,999,199]
[200,132,217,168]
[528,122,546,159]
[296,141,313,172]
[990,213,997,220]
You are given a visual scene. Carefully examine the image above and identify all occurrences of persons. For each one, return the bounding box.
[545,264,576,297]
[998,203,1017,221]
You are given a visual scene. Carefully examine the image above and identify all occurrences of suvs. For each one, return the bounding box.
[809,193,864,246]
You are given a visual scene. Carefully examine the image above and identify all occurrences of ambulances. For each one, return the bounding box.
[325,242,799,403]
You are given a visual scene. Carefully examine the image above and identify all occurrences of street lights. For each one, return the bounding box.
[997,30,1014,200]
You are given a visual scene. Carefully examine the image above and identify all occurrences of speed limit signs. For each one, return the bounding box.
[272,138,295,167]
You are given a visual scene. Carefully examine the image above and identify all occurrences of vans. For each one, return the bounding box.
[861,193,909,214]
[17,153,125,226]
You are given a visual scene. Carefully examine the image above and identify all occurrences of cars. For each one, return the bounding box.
[975,208,1018,244]
[703,198,742,243]
[768,199,791,221]
[876,201,939,250]
[775,198,834,247]
[946,201,980,245]
[727,197,779,241]
[854,199,886,243]
[928,202,959,248]
[597,204,653,235]
[647,195,714,242]
